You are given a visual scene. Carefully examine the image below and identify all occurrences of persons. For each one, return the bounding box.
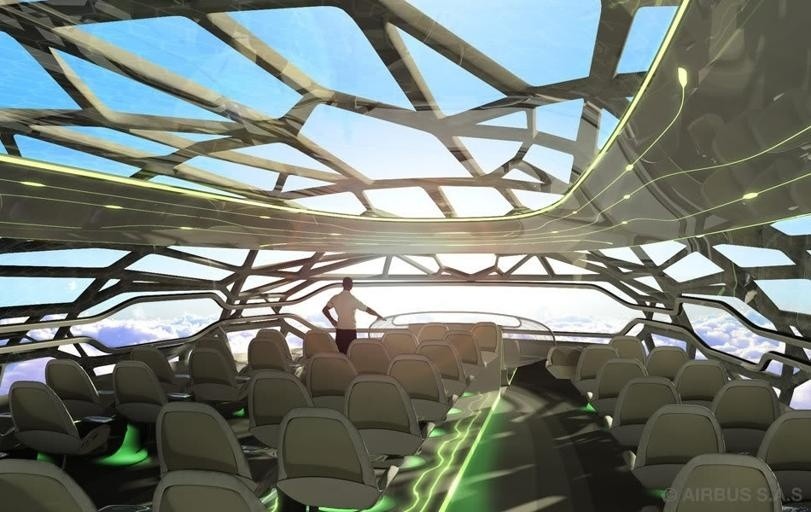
[322,277,387,354]
